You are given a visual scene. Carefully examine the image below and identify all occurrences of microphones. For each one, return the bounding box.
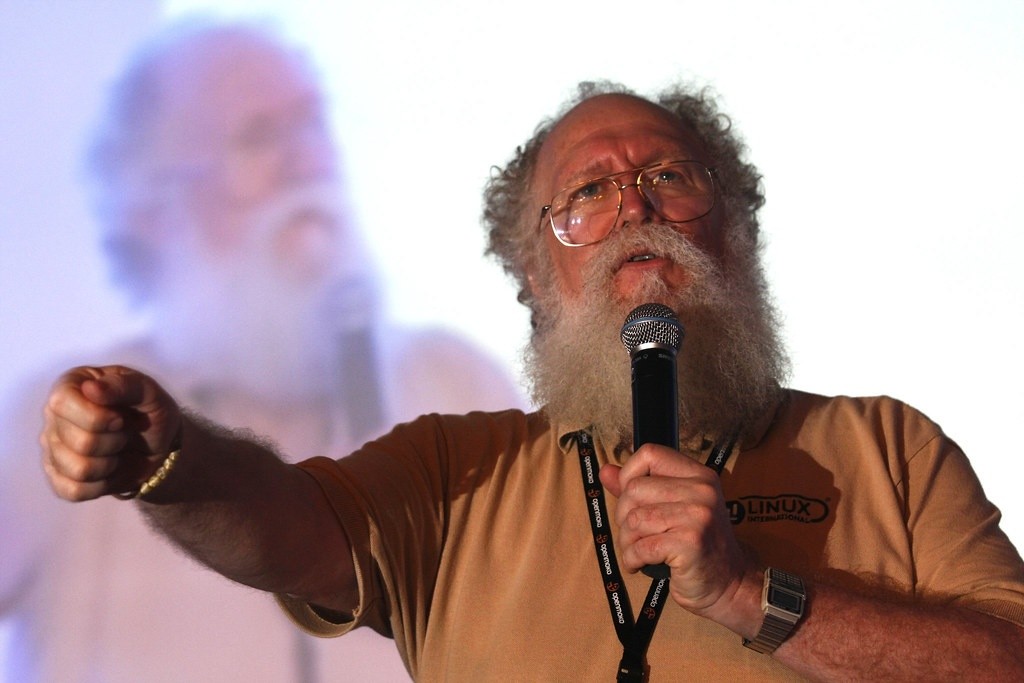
[619,302,687,581]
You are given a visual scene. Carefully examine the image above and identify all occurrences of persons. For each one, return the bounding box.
[39,82,1024,682]
[2,13,530,683]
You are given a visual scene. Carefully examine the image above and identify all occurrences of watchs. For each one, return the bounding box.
[742,566,807,654]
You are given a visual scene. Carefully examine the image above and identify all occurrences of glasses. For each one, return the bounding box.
[535,159,721,247]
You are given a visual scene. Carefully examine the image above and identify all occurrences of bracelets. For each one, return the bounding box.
[112,419,184,501]
